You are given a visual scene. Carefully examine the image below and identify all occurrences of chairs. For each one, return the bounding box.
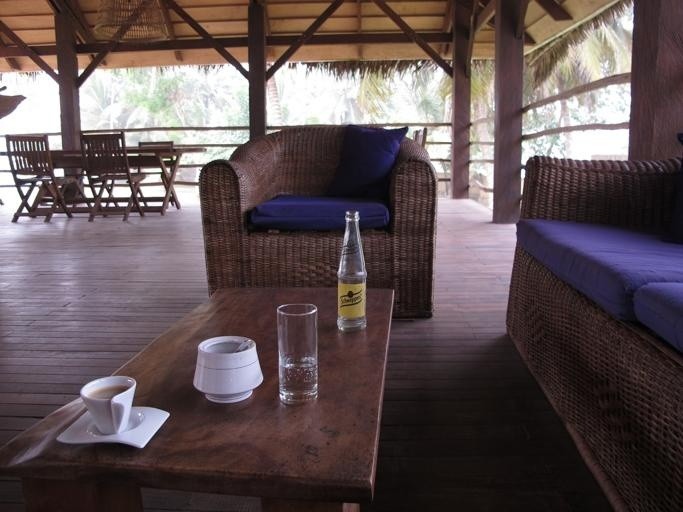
[6,135,92,224]
[79,132,146,222]
[135,141,173,207]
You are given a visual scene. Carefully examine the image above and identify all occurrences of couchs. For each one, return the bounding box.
[199,124,437,318]
[506,157,682,511]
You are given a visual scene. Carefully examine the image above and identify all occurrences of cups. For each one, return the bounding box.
[275,302,320,406]
[79,375,138,436]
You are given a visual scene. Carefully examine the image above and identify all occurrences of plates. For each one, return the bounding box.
[55,406,171,449]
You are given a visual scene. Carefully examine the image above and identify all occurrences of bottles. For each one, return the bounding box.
[337,211,367,332]
